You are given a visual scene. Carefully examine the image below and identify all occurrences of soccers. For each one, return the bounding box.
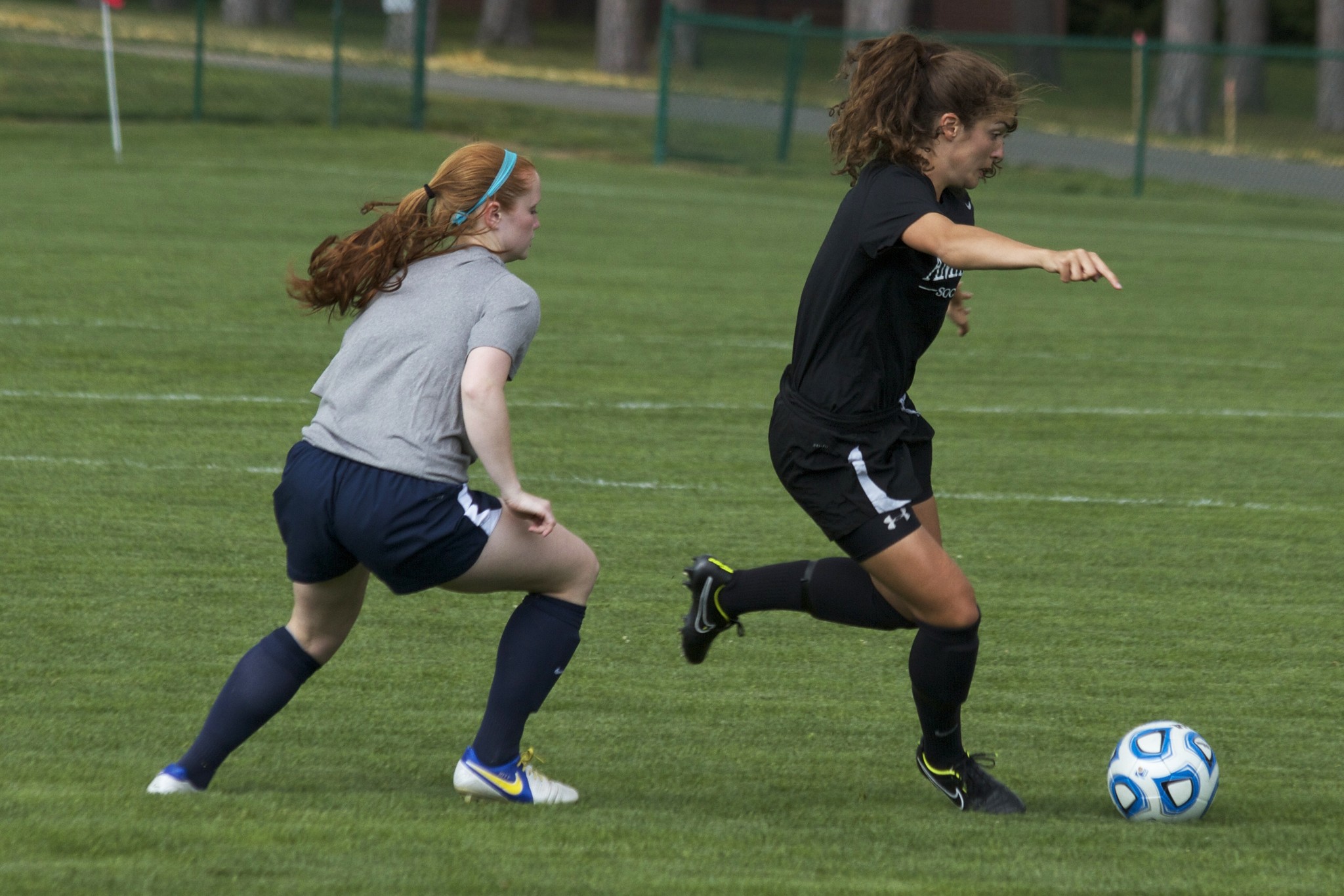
[1106,721,1219,822]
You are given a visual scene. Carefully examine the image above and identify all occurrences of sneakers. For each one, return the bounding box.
[145,763,201,795]
[915,736,1024,817]
[453,746,579,807]
[679,555,745,665]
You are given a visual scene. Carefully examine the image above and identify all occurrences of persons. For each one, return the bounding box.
[147,140,601,806]
[680,30,1128,814]
